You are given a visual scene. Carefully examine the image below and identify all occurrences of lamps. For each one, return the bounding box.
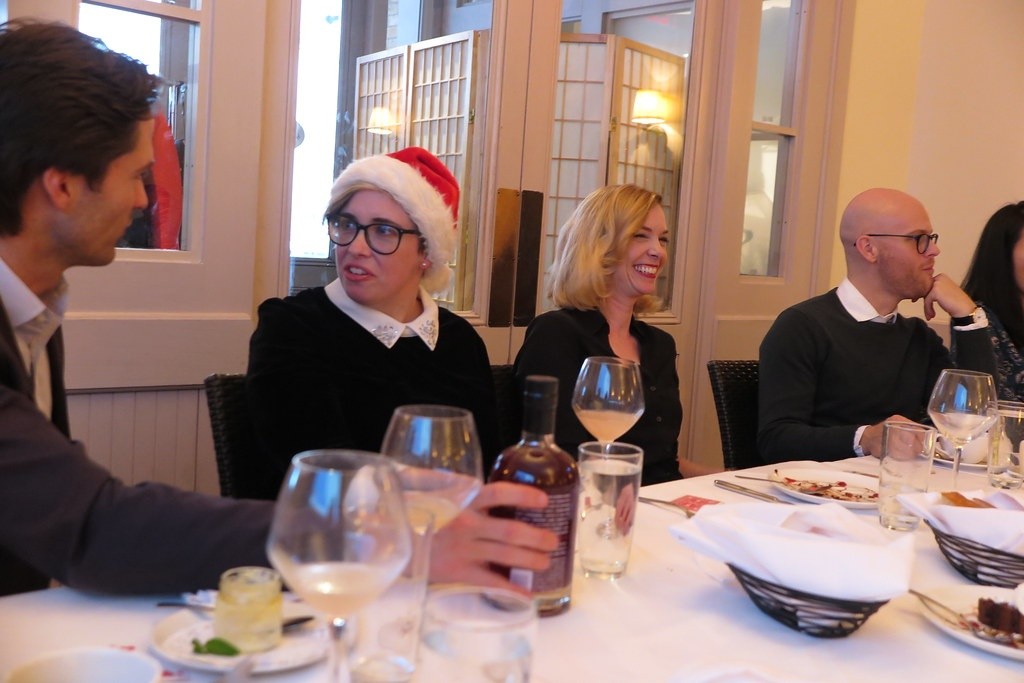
[631,89,668,127]
[367,107,394,136]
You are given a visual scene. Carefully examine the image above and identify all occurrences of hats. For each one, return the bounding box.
[325,147,461,293]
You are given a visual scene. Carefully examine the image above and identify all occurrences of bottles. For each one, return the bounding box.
[480,374,581,618]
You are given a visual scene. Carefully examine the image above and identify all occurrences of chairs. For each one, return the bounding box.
[708,358,763,469]
[203,372,250,498]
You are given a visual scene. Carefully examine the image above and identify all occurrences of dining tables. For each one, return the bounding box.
[0,455,1024,683]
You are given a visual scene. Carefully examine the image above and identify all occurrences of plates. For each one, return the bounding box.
[917,584,1024,661]
[148,603,331,675]
[920,452,987,470]
[767,467,880,510]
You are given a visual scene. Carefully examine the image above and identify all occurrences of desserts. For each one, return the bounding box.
[977,582,1024,642]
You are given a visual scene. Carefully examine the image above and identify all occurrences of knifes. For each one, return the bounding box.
[716,480,794,505]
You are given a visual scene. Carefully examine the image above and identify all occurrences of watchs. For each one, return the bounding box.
[951,305,988,326]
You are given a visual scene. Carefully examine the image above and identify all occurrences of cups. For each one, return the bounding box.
[878,420,938,534]
[935,431,989,463]
[343,501,436,683]
[212,565,282,653]
[576,441,645,584]
[414,583,539,683]
[3,646,163,683]
[987,401,1024,490]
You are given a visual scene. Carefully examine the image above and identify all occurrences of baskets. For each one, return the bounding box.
[727,563,888,639]
[925,520,1024,588]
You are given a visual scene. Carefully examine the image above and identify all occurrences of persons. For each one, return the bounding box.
[512,184,685,486]
[759,188,999,465]
[241,146,535,505]
[1,19,560,601]
[951,201,1024,402]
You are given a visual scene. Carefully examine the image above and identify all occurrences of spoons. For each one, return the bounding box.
[907,589,1022,644]
[156,601,315,629]
[734,475,832,495]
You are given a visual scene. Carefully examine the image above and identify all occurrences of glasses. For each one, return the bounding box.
[854,234,938,254]
[328,213,420,255]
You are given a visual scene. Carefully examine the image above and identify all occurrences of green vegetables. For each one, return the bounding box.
[192,637,240,656]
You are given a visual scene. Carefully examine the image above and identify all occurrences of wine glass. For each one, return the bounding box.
[264,448,413,683]
[571,355,646,460]
[374,403,484,683]
[926,369,999,491]
